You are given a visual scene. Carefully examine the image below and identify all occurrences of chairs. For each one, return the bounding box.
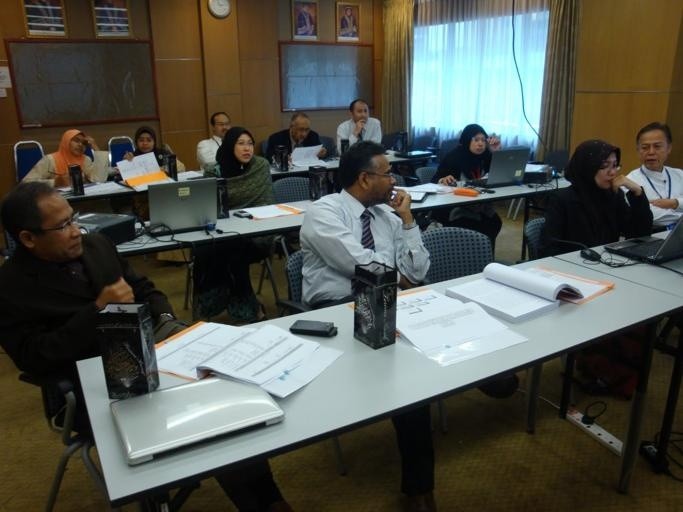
[417,227,538,435]
[13,140,45,181]
[108,135,136,168]
[1,255,222,512]
[526,216,649,422]
[260,140,269,155]
[83,143,95,162]
[392,172,417,186]
[267,178,321,299]
[288,248,448,478]
[440,139,462,161]
[412,135,435,151]
[416,167,437,185]
[319,136,335,157]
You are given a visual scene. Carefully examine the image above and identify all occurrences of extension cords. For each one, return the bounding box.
[566,408,623,456]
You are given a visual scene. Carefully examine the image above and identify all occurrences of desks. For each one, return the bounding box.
[82,161,573,255]
[75,226,683,511]
[58,144,437,201]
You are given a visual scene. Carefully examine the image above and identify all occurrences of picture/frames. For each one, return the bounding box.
[291,1,320,41]
[335,1,362,43]
[21,0,70,39]
[92,0,133,38]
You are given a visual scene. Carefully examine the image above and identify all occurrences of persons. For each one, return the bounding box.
[620,122,683,233]
[192,127,270,321]
[0,182,288,508]
[20,129,109,190]
[337,99,383,157]
[545,140,653,402]
[431,124,502,246]
[123,126,163,166]
[196,112,231,169]
[299,140,520,507]
[266,112,327,164]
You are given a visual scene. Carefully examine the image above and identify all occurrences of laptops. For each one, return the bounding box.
[604,213,683,265]
[465,148,530,188]
[146,177,218,236]
[110,376,285,467]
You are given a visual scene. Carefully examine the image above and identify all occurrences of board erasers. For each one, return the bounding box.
[20,123,42,128]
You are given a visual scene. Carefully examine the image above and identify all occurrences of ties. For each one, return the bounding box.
[351,210,375,294]
[294,140,299,150]
[358,129,363,144]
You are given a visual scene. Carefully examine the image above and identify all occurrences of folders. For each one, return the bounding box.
[243,204,306,220]
[348,288,528,367]
[523,265,615,306]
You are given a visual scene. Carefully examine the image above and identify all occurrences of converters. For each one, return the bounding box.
[639,441,670,471]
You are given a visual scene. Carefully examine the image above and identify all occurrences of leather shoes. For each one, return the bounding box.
[405,491,436,511]
[477,373,520,397]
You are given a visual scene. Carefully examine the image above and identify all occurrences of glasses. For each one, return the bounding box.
[363,169,393,178]
[234,140,253,145]
[599,165,621,172]
[472,136,487,142]
[33,210,80,230]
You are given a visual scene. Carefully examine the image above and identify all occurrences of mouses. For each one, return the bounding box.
[581,248,601,261]
[448,180,457,187]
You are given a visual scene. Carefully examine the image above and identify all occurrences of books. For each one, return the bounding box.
[446,262,584,323]
[242,199,314,220]
[396,288,528,368]
[391,181,454,204]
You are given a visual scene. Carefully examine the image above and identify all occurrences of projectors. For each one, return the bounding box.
[72,210,136,245]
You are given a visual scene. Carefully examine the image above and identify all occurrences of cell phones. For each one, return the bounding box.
[290,320,334,336]
[234,210,250,217]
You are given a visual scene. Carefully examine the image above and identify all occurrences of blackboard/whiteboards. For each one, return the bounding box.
[4,37,161,130]
[277,39,377,111]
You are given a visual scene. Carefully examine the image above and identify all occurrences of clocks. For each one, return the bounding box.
[208,0,231,18]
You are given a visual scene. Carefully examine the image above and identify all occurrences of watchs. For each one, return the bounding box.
[401,218,416,230]
